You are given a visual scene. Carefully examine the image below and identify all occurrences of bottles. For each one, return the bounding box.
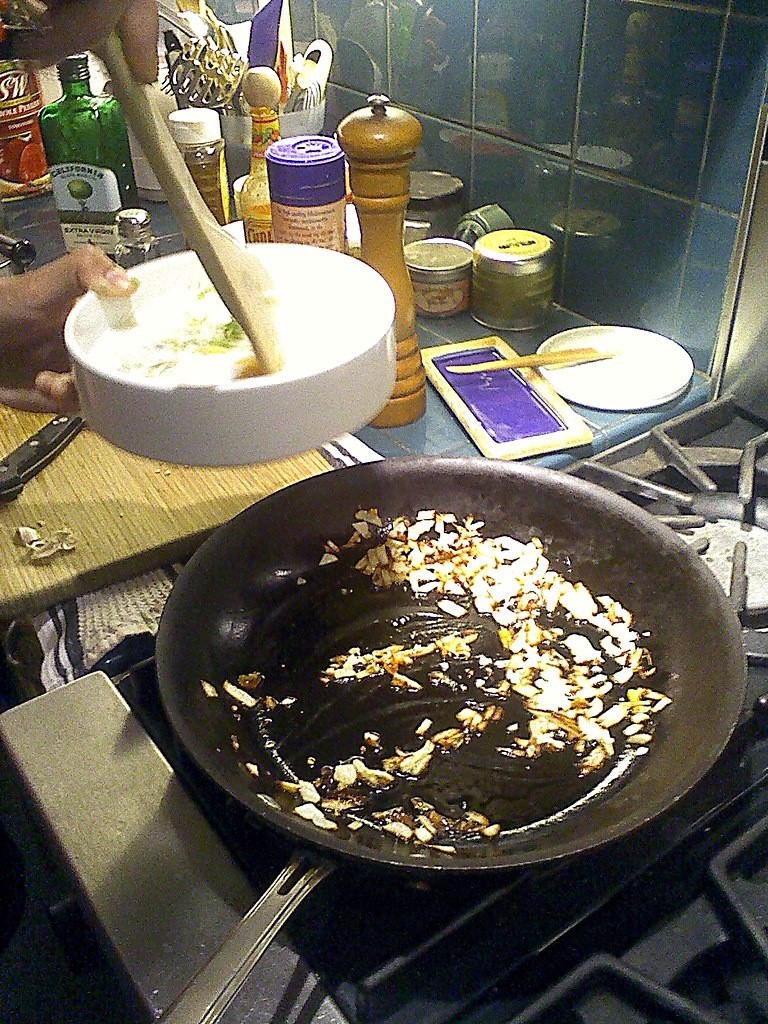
[403,171,464,245]
[265,136,346,252]
[240,107,281,241]
[168,109,228,249]
[36,53,139,264]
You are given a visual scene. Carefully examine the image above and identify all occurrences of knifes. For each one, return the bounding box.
[0,406,85,501]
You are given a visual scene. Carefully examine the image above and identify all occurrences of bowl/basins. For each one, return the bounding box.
[64,239,395,467]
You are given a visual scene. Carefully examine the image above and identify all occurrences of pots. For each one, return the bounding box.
[154,456,748,1024]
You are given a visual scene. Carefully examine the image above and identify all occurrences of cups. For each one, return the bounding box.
[221,98,325,222]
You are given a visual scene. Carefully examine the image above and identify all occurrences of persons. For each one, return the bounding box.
[0,0,161,417]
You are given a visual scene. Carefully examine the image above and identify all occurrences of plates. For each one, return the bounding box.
[537,324,693,410]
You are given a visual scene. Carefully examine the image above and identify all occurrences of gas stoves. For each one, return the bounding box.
[0,379,768,1024]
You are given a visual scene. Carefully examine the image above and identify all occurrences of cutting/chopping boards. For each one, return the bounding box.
[0,404,337,630]
